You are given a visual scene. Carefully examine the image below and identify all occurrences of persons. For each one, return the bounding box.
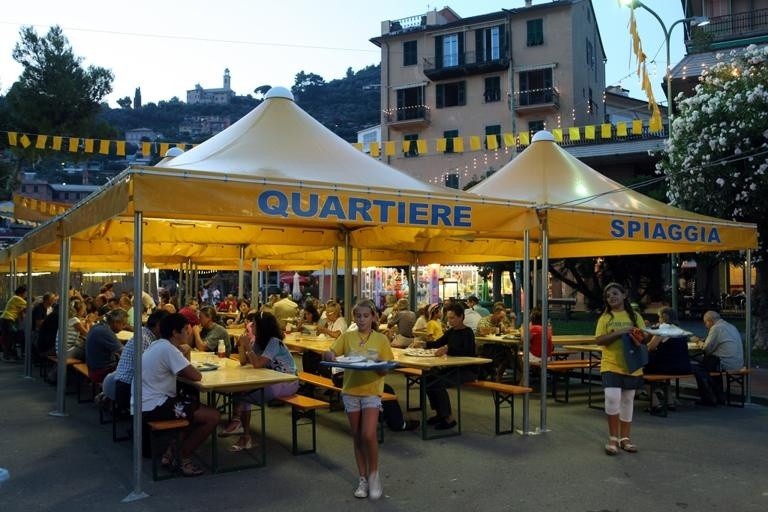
[634,306,746,417]
[378,295,552,428]
[332,319,420,431]
[595,283,647,455]
[321,300,392,500]
[1,281,348,477]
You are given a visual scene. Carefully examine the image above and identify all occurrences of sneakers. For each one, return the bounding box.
[161,452,177,465]
[354,475,369,499]
[370,473,384,500]
[178,458,205,476]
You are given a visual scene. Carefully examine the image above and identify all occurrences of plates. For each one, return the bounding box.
[644,323,693,338]
[335,348,389,369]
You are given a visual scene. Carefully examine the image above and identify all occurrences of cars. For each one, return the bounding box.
[258,282,284,303]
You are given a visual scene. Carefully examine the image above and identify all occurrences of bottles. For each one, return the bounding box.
[217,338,227,367]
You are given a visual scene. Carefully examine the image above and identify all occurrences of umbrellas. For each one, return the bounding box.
[292,270,303,301]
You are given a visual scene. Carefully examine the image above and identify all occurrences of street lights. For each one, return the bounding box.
[623,0,712,312]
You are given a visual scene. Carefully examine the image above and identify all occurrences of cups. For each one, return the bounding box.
[294,333,301,343]
[403,341,440,357]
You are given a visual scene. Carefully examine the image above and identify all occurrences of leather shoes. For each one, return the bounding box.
[405,415,458,429]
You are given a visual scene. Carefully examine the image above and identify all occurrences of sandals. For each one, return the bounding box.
[667,401,676,411]
[231,435,254,451]
[621,435,640,453]
[219,421,245,437]
[605,435,620,455]
[644,404,664,414]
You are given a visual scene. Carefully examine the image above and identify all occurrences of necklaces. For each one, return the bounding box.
[358,328,372,346]
